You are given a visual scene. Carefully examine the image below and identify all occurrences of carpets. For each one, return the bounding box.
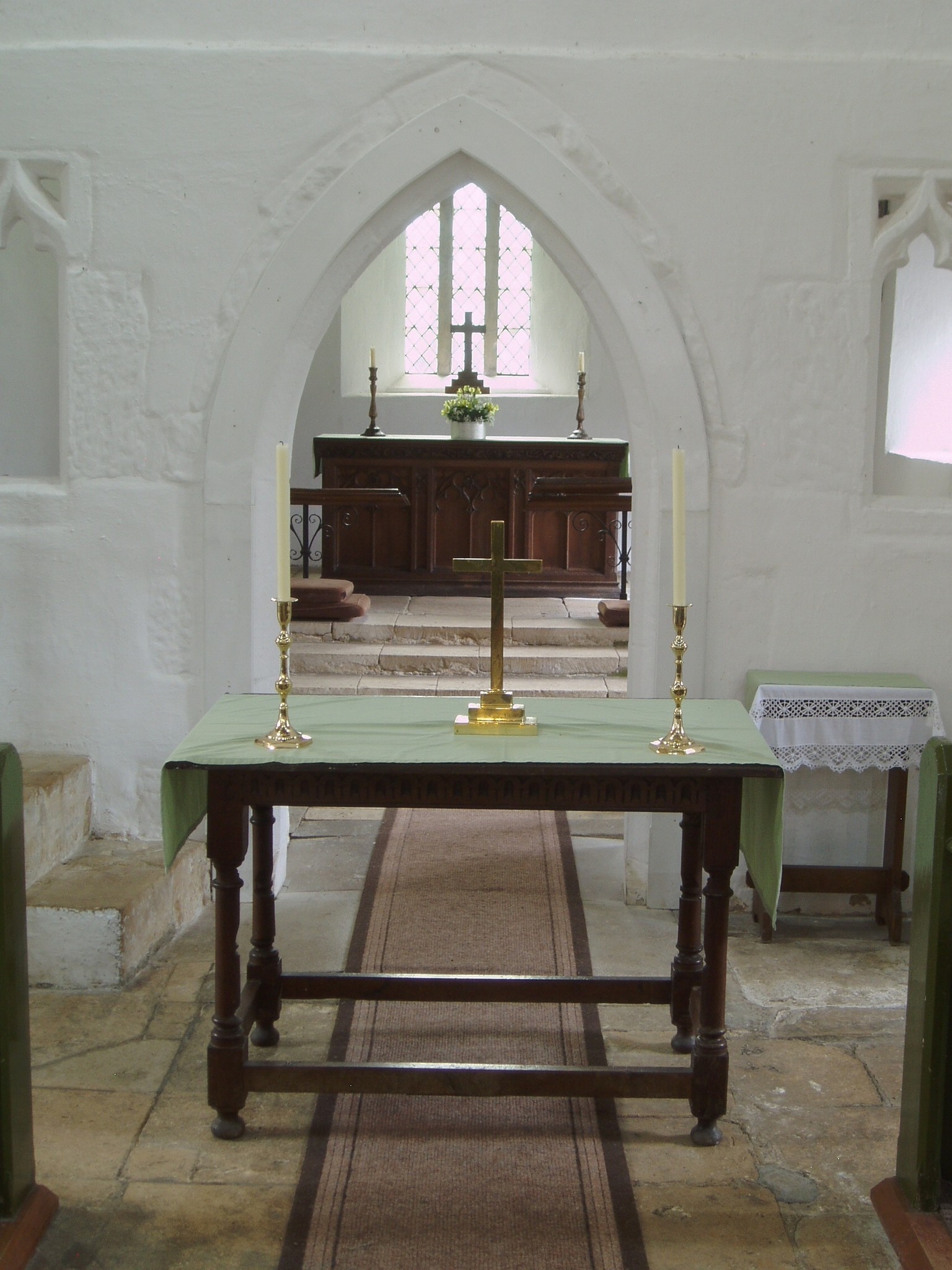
[274,806,652,1270]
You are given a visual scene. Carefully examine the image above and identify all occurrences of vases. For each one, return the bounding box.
[449,418,486,440]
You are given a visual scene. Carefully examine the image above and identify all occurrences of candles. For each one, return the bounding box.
[274,439,293,598]
[369,346,377,368]
[578,350,585,372]
[669,443,690,608]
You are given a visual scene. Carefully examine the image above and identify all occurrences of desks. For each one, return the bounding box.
[738,668,947,944]
[312,432,632,599]
[157,688,787,1147]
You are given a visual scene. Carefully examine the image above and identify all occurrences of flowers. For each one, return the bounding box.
[440,384,500,427]
